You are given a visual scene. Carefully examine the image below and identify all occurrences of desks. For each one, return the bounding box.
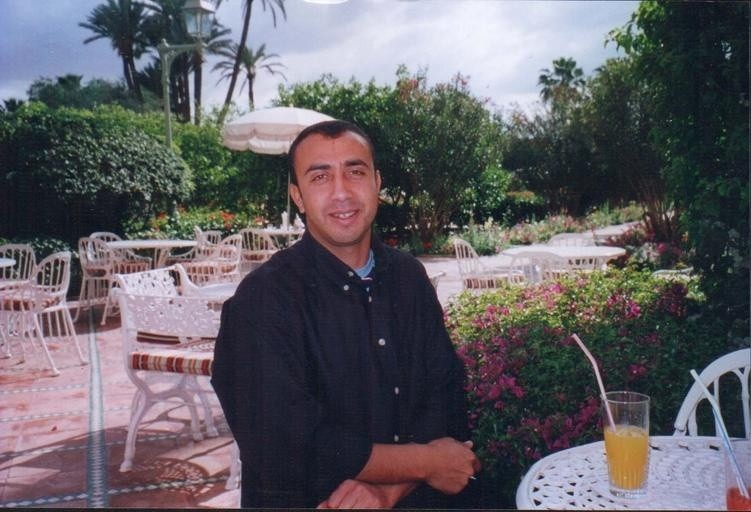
[511,428,749,512]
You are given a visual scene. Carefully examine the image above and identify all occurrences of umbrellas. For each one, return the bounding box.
[219,101,341,243]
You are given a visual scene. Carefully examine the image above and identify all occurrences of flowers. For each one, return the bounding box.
[440,230,694,493]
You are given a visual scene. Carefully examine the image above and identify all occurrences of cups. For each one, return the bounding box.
[265,222,305,232]
[722,437,751,511]
[600,389,652,499]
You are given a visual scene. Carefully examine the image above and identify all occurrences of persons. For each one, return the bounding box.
[212,123,477,510]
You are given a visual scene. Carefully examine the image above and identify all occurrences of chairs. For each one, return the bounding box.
[662,341,749,442]
[416,223,695,295]
[2,223,304,493]
